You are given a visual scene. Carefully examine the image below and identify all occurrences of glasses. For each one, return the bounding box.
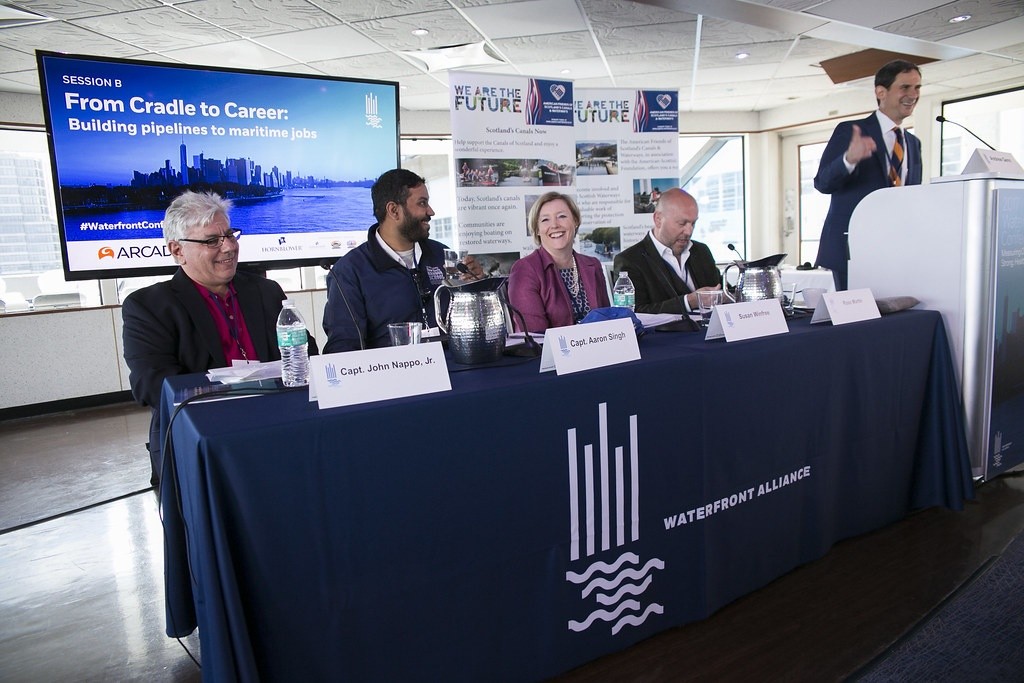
[177,228,242,249]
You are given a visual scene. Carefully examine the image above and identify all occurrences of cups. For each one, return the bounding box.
[443,248,468,275]
[802,288,828,307]
[695,290,723,327]
[386,322,423,347]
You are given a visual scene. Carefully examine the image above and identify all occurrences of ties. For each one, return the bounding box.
[888,127,904,187]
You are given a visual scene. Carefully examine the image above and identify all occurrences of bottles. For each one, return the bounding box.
[275,299,310,387]
[612,271,636,314]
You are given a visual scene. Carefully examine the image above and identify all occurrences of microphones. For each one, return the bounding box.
[639,247,702,333]
[936,116,997,152]
[728,243,744,262]
[320,258,365,352]
[457,262,544,357]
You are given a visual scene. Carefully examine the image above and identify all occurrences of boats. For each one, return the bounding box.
[460,179,496,186]
[539,164,573,186]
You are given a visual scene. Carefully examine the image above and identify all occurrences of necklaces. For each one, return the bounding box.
[684,264,688,284]
[562,255,578,298]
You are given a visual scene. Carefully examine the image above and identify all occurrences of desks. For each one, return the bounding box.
[159,306,976,683]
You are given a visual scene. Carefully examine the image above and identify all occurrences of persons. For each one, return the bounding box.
[122,192,319,480]
[614,188,745,314]
[463,162,494,183]
[508,191,612,333]
[323,168,488,353]
[814,60,922,292]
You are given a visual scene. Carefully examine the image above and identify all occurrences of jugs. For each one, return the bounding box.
[722,252,788,304]
[434,275,509,366]
